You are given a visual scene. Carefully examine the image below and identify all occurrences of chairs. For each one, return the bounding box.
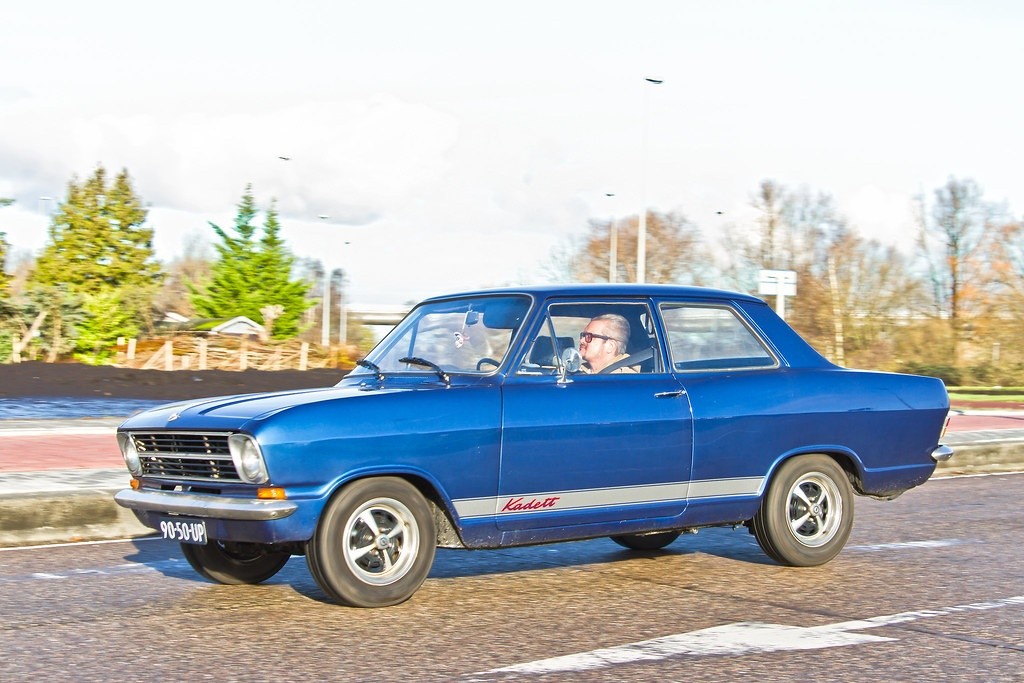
[529,336,574,366]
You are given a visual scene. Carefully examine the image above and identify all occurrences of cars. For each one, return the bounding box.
[113,285,953,608]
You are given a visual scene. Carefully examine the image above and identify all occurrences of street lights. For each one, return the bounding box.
[316,215,331,349]
[603,191,619,283]
[24,194,53,285]
[340,240,355,347]
[278,155,295,277]
[636,76,664,286]
[713,211,725,293]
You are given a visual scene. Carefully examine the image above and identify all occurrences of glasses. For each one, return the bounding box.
[580,332,609,343]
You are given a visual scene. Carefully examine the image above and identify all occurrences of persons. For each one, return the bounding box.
[578,314,637,373]
